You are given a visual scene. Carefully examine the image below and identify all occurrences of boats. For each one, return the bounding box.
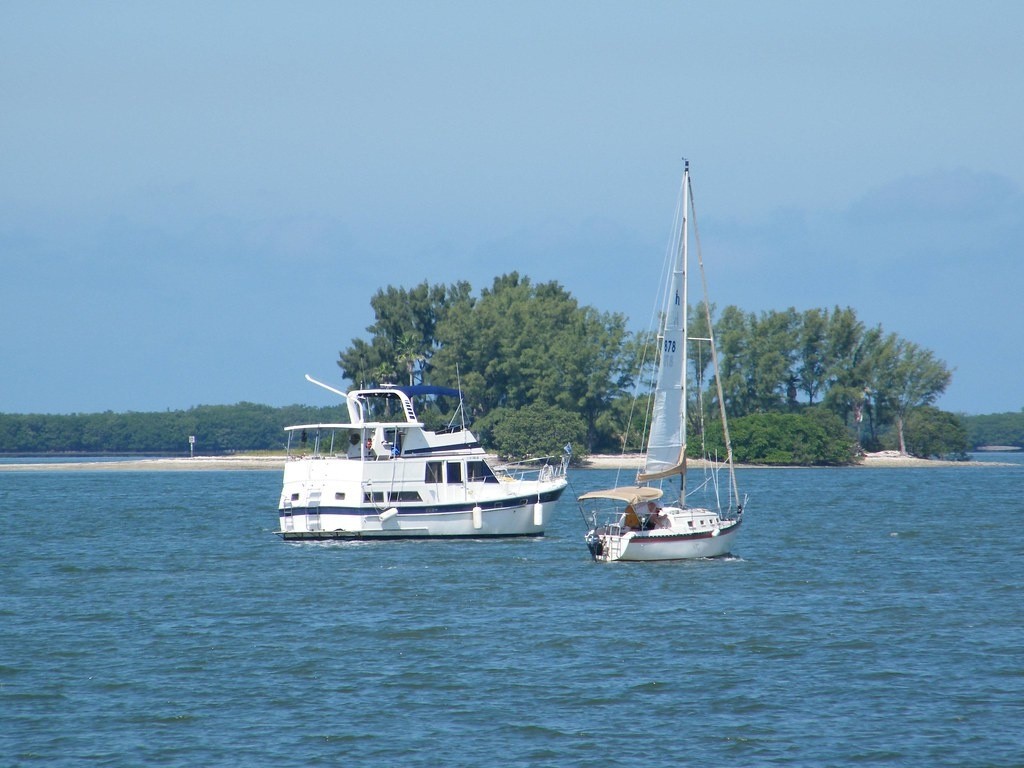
[270,359,572,542]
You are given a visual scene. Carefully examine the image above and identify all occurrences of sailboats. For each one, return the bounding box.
[577,156,753,564]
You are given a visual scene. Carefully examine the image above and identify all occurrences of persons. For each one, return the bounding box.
[645,502,671,530]
